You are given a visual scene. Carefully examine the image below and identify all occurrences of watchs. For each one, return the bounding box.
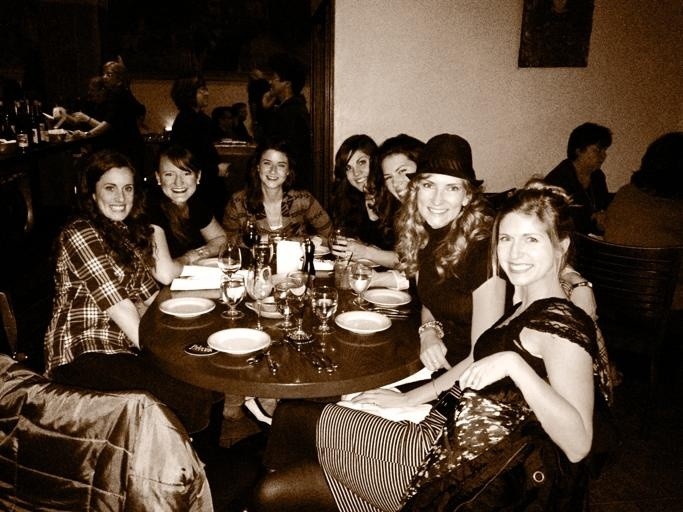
[85,131,91,137]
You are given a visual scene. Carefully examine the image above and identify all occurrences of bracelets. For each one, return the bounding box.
[87,116,91,124]
[418,321,445,339]
[432,378,440,401]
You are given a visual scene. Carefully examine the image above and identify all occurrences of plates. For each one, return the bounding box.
[207,327,271,358]
[158,296,215,319]
[336,310,392,337]
[361,287,413,309]
[47,129,68,135]
[220,139,247,145]
[245,300,292,318]
[195,256,235,268]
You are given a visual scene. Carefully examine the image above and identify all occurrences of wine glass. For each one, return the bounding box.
[253,241,276,265]
[272,273,294,344]
[219,246,243,280]
[242,223,260,268]
[245,264,273,341]
[289,272,314,345]
[333,226,343,235]
[221,280,246,322]
[350,262,371,307]
[308,286,338,372]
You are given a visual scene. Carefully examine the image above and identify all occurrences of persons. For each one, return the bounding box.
[334,133,510,427]
[259,59,311,160]
[332,133,425,271]
[247,66,270,138]
[142,143,229,286]
[592,130,683,322]
[42,150,225,478]
[326,135,379,242]
[258,183,622,512]
[71,59,143,171]
[170,70,231,192]
[544,120,612,240]
[222,139,337,249]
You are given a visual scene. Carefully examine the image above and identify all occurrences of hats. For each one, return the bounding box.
[413,134,485,184]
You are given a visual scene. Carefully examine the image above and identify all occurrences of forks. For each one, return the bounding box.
[354,302,410,320]
[299,343,340,375]
[249,337,286,376]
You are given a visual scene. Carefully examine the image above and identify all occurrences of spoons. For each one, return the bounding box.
[335,252,353,286]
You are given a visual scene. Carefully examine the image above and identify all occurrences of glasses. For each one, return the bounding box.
[269,77,281,81]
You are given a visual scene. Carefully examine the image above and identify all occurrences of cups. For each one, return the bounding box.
[258,302,278,312]
[335,262,354,291]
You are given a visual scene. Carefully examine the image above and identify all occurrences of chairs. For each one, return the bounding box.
[573,232,682,441]
